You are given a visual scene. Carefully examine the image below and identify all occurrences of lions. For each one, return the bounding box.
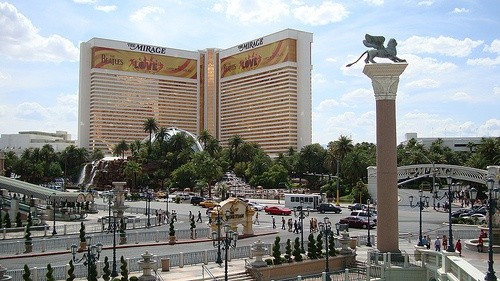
[346,34,406,67]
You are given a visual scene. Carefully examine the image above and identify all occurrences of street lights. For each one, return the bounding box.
[292,200,311,253]
[101,211,128,278]
[211,224,239,281]
[165,187,170,217]
[366,198,373,247]
[45,192,63,234]
[145,190,154,227]
[208,205,231,263]
[408,190,430,246]
[70,236,103,281]
[318,216,340,281]
[433,174,463,252]
[484,177,500,281]
[101,190,117,230]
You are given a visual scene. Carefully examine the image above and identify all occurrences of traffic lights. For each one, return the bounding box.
[328,174,332,180]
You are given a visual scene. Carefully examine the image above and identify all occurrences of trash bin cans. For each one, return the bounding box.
[350,238,357,248]
[161,258,169,271]
[65,212,70,220]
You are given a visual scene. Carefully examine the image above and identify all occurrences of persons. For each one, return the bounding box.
[253,212,260,225]
[288,218,293,232]
[480,229,488,238]
[422,235,431,250]
[477,237,483,252]
[434,236,441,251]
[271,215,276,228]
[442,235,447,250]
[309,217,319,233]
[154,209,178,223]
[196,211,203,223]
[280,217,286,229]
[455,239,461,254]
[294,219,301,234]
[189,210,194,222]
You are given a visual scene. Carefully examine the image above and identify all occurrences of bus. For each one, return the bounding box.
[285,194,328,211]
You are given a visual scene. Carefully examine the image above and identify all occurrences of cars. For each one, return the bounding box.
[173,193,191,200]
[189,195,222,207]
[452,205,494,224]
[246,201,292,215]
[339,202,377,230]
[317,203,342,214]
